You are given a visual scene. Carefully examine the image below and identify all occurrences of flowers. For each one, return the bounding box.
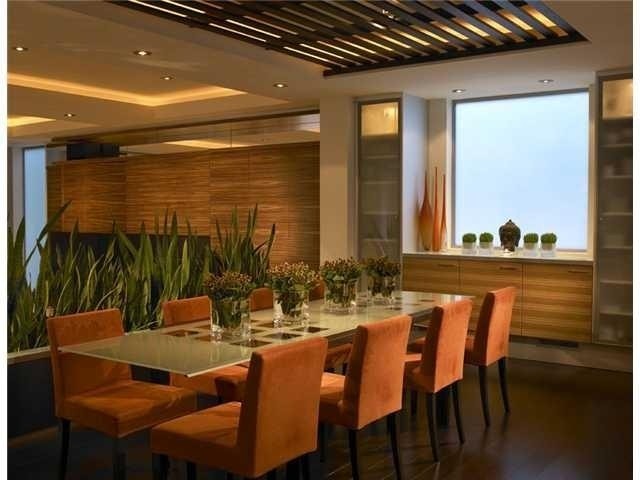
[204,256,401,329]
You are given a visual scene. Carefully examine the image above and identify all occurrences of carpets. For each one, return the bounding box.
[505,335,632,375]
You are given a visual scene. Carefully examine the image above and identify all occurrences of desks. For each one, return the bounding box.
[56,289,477,474]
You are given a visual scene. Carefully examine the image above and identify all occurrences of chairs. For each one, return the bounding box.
[148,335,330,477]
[319,312,414,478]
[308,281,325,301]
[45,307,199,479]
[161,294,249,401]
[412,286,519,428]
[403,299,473,462]
[250,288,274,329]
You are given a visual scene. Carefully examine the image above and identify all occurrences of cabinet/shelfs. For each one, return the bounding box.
[592,69,632,347]
[403,256,592,344]
[357,97,403,291]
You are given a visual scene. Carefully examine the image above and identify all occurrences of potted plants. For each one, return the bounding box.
[524,233,557,250]
[463,233,493,248]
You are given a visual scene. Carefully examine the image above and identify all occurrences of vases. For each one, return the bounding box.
[418,167,448,251]
